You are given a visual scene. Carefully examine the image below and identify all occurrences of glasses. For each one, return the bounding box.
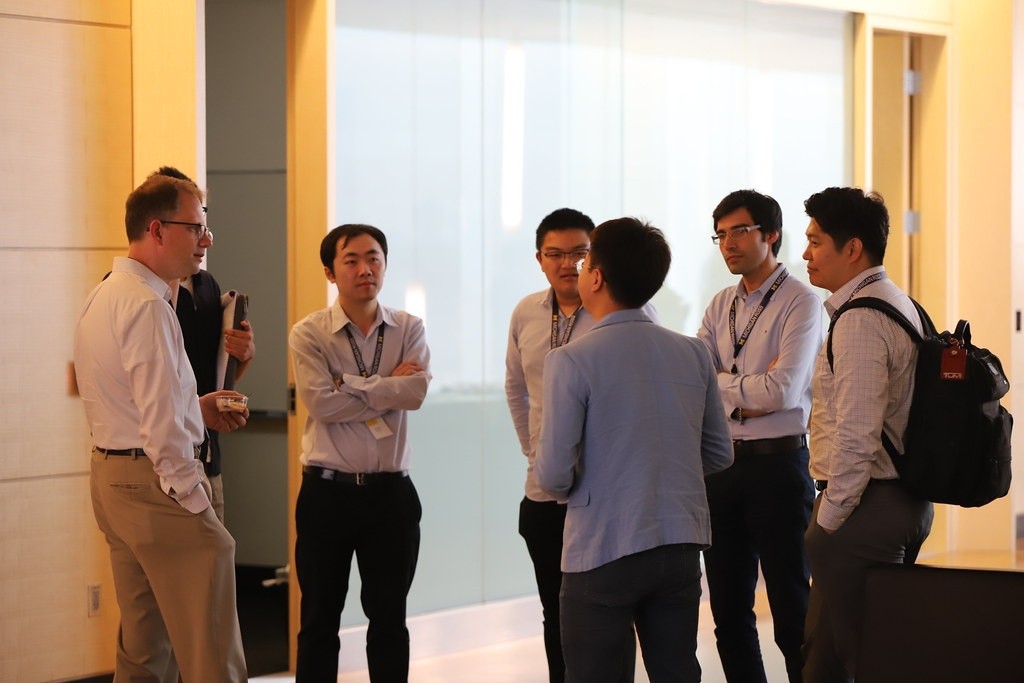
[539,248,590,263]
[574,257,609,284]
[711,223,763,245]
[147,217,213,241]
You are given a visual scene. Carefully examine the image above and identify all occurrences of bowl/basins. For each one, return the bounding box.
[215,395,249,414]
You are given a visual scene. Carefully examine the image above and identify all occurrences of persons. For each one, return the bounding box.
[73,173,249,683]
[288,223,435,683]
[101,167,257,524]
[505,205,664,683]
[532,217,735,683]
[695,188,823,683]
[798,187,935,683]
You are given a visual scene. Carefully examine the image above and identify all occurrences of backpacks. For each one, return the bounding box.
[827,295,1013,508]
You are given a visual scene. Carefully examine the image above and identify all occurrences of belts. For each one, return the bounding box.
[815,476,882,491]
[304,461,410,486]
[94,446,148,457]
[733,431,805,456]
